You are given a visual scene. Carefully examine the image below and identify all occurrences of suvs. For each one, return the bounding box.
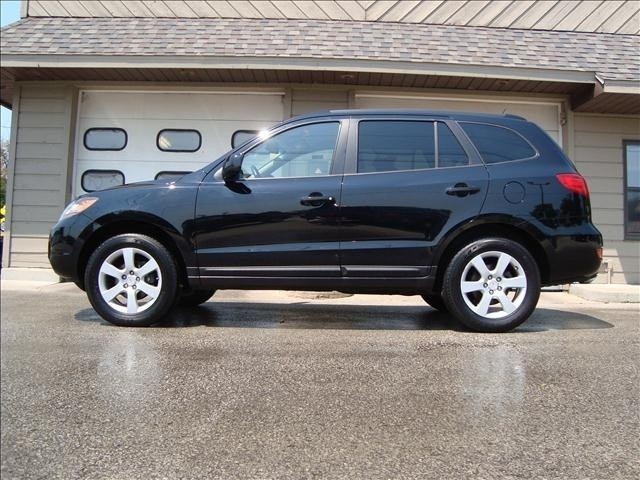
[48,112,603,332]
